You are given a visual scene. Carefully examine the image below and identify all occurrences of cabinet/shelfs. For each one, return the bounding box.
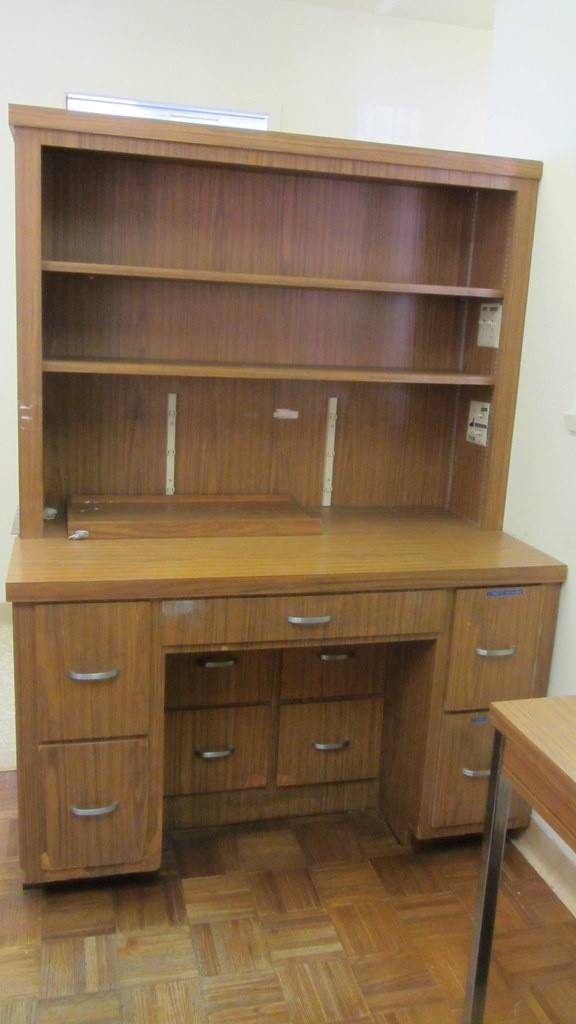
[5,104,568,891]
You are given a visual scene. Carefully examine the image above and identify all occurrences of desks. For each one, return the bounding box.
[462,693,576,1024]
